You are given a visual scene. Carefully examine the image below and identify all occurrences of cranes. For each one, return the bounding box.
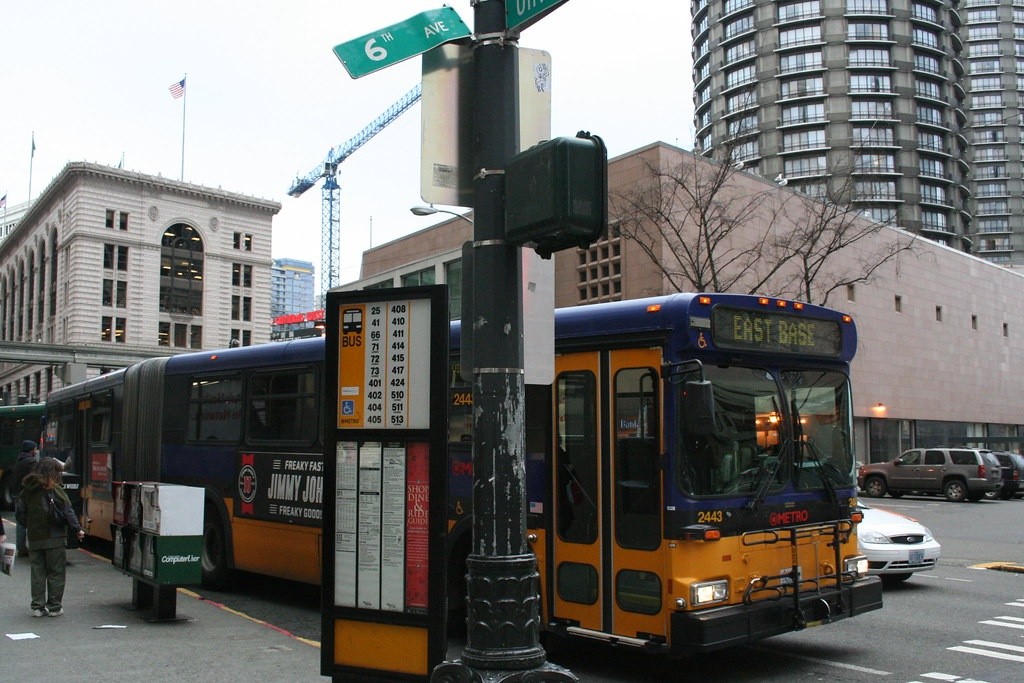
[286,77,425,312]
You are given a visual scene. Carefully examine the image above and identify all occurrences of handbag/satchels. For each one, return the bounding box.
[49,480,68,538]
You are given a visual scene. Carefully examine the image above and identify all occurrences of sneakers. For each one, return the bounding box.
[48,607,64,617]
[32,607,49,617]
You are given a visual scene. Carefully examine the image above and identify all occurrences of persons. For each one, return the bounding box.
[0,464,6,543]
[14,458,85,616]
[10,440,39,558]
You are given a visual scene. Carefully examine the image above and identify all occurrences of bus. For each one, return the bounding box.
[41,291,884,661]
[0,402,49,512]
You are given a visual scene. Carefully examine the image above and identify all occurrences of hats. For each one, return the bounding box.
[22,440,37,454]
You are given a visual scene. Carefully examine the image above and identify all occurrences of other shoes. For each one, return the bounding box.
[18,547,30,558]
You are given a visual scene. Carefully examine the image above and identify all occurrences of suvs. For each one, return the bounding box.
[984,449,1024,500]
[857,445,1005,503]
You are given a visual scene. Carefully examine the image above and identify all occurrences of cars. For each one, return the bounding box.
[856,498,941,584]
